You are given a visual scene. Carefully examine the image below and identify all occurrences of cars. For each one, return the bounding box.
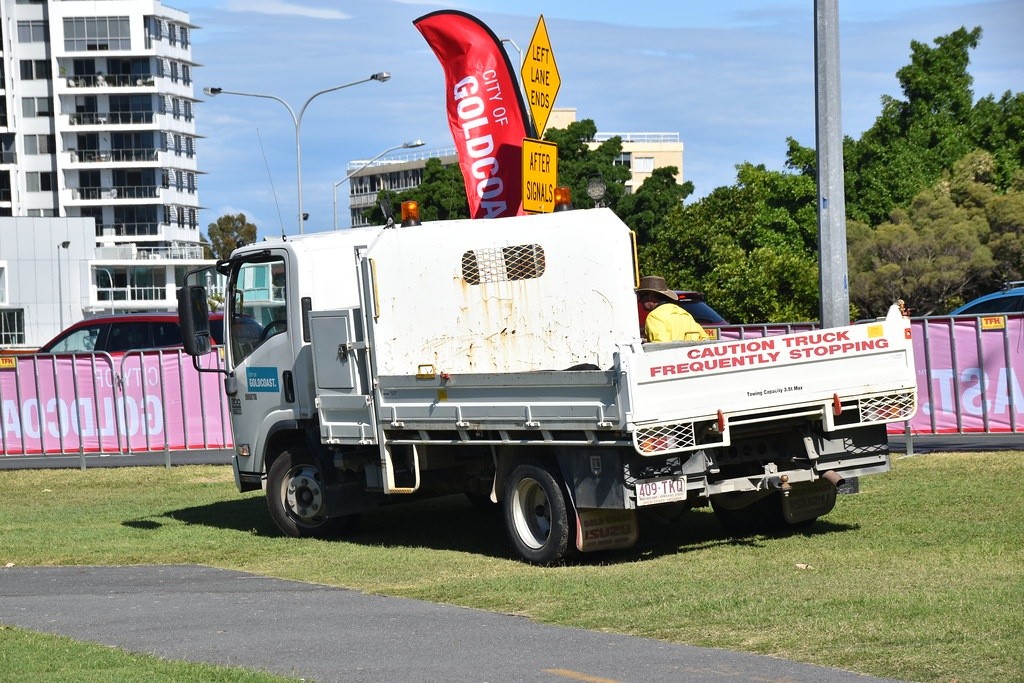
[32,309,270,358]
[947,287,1023,315]
[668,289,729,329]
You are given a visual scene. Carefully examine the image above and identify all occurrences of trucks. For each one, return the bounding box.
[174,178,918,569]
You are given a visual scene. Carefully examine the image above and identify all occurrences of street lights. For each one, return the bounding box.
[56,240,73,338]
[332,139,427,232]
[202,71,392,235]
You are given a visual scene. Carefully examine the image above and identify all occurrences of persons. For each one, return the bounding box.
[636,276,710,344]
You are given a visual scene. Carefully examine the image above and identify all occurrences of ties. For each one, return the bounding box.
[637,276,679,301]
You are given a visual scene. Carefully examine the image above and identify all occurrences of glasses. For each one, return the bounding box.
[636,292,649,301]
